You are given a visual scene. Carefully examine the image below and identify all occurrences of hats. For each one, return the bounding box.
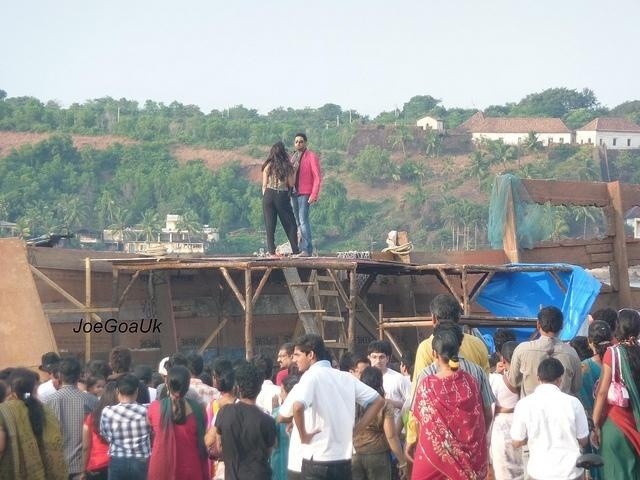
[38,351,64,373]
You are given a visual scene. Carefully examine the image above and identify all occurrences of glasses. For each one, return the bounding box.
[294,139,305,144]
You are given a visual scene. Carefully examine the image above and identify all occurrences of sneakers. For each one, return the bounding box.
[291,252,311,257]
[270,253,284,258]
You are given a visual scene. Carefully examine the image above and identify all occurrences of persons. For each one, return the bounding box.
[290,133,320,256]
[259,140,301,256]
[0,293,639,480]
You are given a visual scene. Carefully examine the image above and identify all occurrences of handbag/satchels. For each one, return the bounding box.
[589,377,631,410]
